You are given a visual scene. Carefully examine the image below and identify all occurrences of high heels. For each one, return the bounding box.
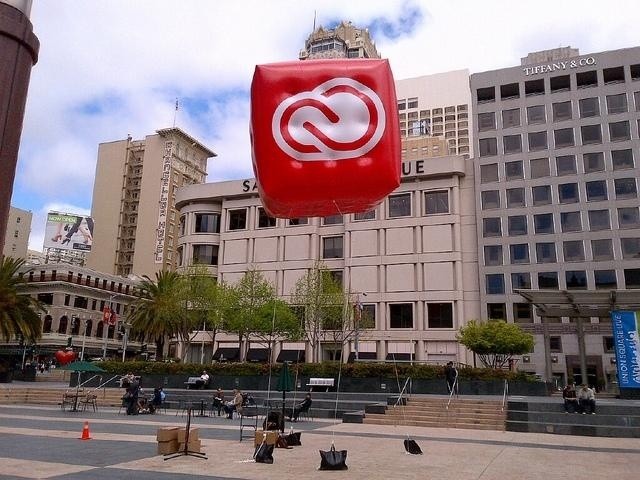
[78,421,90,440]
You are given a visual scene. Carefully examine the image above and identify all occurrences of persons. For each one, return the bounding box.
[51,222,92,244]
[597,375,605,391]
[578,383,596,415]
[38,359,52,373]
[121,371,166,415]
[213,384,224,416]
[287,393,313,422]
[442,361,456,394]
[195,370,210,389]
[61,217,94,246]
[223,388,244,419]
[563,384,579,414]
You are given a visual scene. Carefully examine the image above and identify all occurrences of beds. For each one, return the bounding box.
[54,360,107,409]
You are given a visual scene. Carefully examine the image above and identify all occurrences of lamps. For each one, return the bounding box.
[176,399,224,418]
[117,395,167,416]
[60,393,98,413]
[239,407,258,440]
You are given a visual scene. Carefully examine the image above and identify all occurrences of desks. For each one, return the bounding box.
[355,292,368,359]
[103,291,136,361]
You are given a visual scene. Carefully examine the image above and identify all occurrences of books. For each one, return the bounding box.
[255,430,278,448]
[156,427,202,455]
